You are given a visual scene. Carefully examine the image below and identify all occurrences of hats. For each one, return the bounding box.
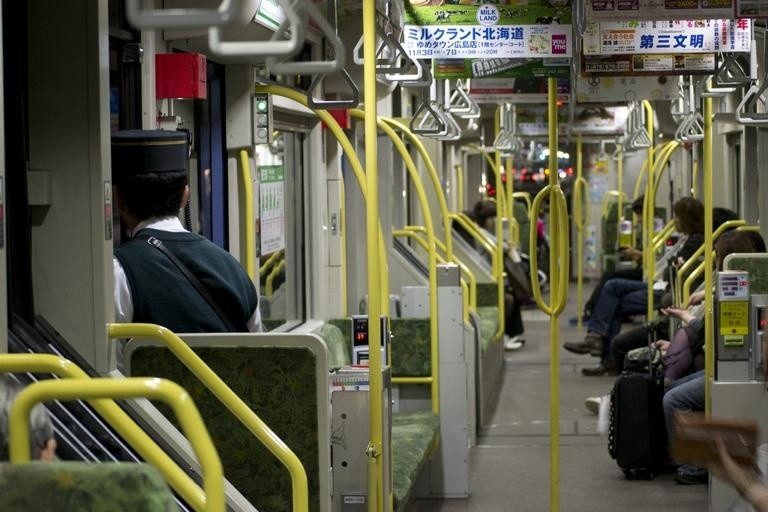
[110,130,188,173]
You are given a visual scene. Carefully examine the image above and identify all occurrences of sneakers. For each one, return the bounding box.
[585,396,606,413]
[582,361,616,377]
[570,311,588,322]
[503,334,525,350]
[563,335,602,355]
[676,465,708,485]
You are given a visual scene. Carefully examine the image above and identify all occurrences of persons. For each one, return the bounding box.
[561,194,766,488]
[697,436,768,510]
[0,370,58,461]
[113,125,264,347]
[466,201,526,350]
[531,205,545,244]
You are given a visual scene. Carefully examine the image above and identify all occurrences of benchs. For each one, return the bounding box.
[311,318,440,510]
[477,282,499,348]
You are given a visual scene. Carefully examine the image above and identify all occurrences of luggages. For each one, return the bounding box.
[608,323,662,480]
